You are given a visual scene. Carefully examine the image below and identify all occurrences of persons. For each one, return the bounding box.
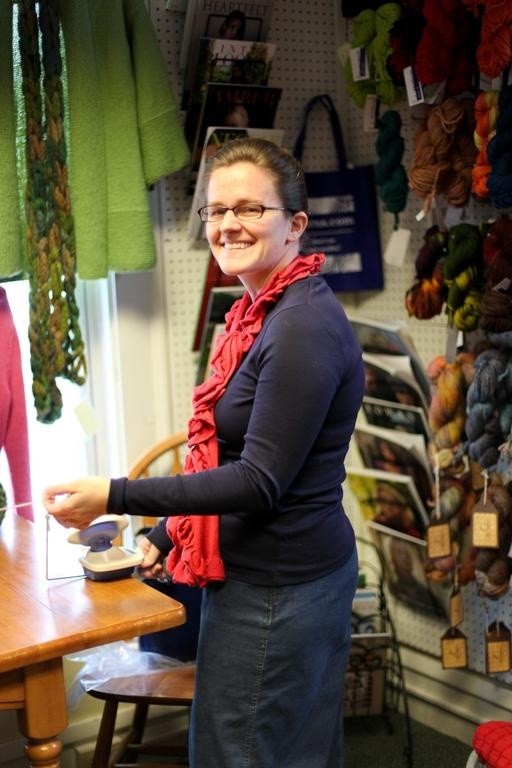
[42,136,365,768]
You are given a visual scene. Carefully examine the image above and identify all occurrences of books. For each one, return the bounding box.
[345,312,448,623]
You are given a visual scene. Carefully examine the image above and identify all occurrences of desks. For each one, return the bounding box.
[2,508,186,768]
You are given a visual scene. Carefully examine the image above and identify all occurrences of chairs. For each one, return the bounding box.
[468,721,512,768]
[75,430,196,767]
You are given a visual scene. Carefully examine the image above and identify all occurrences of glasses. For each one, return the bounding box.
[197,202,298,223]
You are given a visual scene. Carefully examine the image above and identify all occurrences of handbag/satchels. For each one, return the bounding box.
[293,93,384,292]
